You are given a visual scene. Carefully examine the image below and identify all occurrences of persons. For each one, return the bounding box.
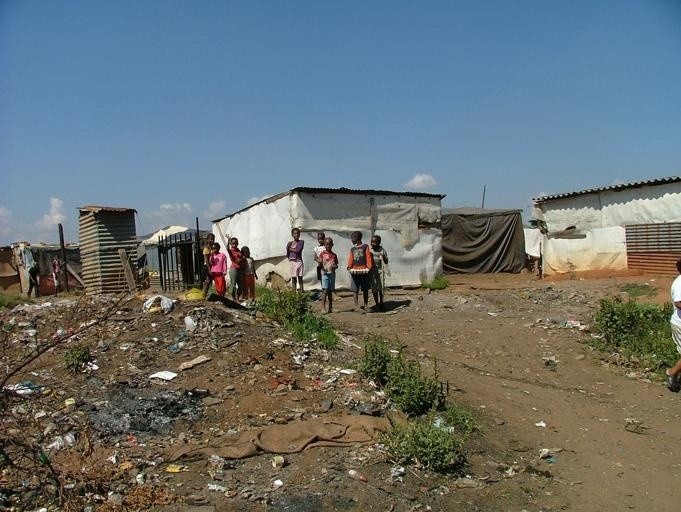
[208,243,228,297]
[313,232,327,282]
[286,228,306,293]
[346,231,375,314]
[27,261,42,297]
[51,252,66,298]
[369,235,389,308]
[224,232,247,301]
[199,233,216,300]
[240,246,259,300]
[315,237,339,315]
[665,257,681,394]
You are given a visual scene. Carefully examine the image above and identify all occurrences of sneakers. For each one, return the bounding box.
[328,307,340,313]
[354,307,373,315]
[320,309,328,315]
